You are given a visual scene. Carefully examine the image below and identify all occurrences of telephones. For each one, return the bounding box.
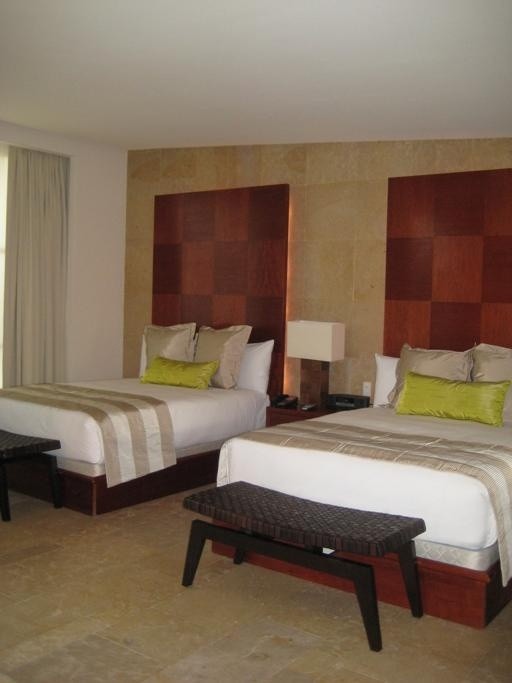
[271,394,297,409]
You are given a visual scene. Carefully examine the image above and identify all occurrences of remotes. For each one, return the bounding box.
[301,402,317,410]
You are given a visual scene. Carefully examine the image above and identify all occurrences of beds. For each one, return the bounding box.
[212,405,512,629]
[0,375,290,515]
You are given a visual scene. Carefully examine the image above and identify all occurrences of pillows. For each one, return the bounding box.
[238,339,274,396]
[388,341,476,408]
[139,353,219,390]
[393,370,511,428]
[195,325,253,391]
[470,343,512,423]
[138,325,147,378]
[146,322,196,369]
[141,334,147,377]
[372,353,400,408]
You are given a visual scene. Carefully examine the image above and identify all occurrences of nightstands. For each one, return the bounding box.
[266,407,346,427]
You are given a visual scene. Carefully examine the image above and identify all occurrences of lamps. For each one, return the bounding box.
[286,320,346,409]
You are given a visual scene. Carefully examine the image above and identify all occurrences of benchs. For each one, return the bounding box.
[182,482,426,651]
[0,430,63,521]
